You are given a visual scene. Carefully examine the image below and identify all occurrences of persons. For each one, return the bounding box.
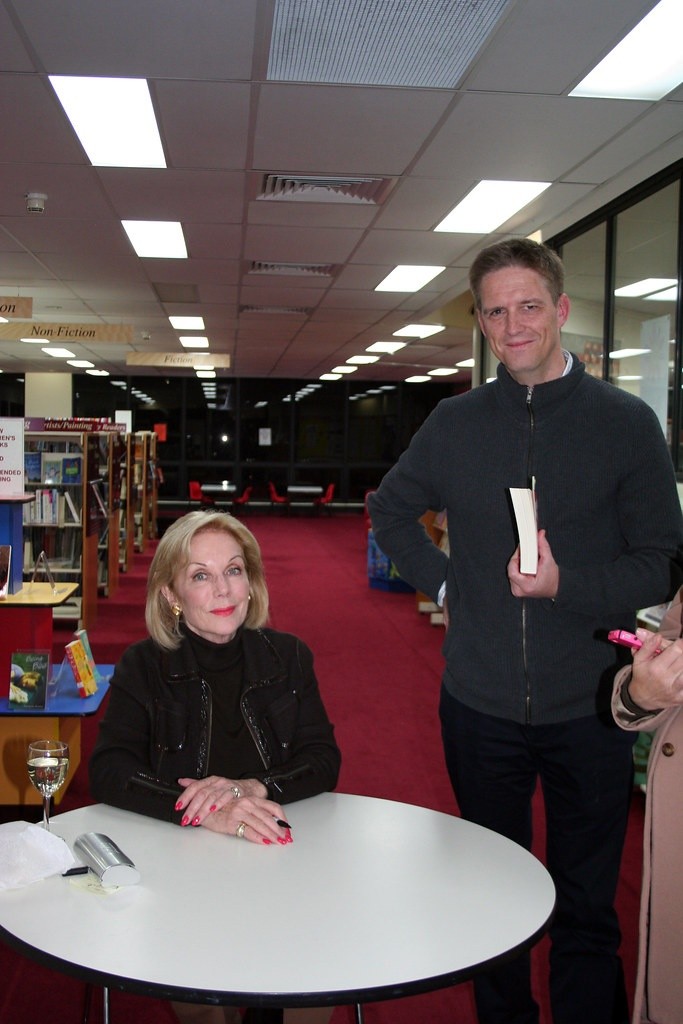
[11,664,42,695]
[370,239,683,1024]
[156,454,163,483]
[88,510,343,1024]
[611,581,683,1024]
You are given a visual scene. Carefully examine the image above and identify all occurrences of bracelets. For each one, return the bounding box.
[619,671,663,717]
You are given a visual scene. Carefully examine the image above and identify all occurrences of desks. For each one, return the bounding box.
[1,790,555,1024]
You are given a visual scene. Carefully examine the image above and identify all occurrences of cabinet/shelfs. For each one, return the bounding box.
[0,419,156,805]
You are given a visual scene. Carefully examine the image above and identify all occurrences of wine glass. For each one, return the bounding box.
[25,740,70,842]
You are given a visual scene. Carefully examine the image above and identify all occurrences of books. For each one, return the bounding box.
[74,627,100,682]
[509,476,539,574]
[8,653,49,711]
[66,639,98,698]
[22,436,153,597]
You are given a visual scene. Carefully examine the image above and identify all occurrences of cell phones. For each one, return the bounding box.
[608,630,661,654]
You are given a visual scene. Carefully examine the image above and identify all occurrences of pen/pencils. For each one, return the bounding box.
[269,814,292,829]
[61,866,91,877]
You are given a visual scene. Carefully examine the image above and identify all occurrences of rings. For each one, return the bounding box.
[236,823,246,837]
[232,787,240,798]
